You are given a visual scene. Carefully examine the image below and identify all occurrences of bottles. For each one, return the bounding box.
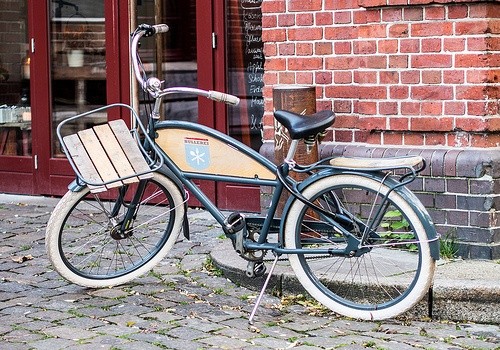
[20,50,30,79]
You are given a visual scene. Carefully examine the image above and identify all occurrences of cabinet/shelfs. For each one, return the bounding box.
[0,61,109,156]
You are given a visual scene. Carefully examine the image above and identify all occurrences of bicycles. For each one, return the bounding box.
[44,24,442,326]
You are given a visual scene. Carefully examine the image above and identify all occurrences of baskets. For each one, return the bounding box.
[56,103,165,191]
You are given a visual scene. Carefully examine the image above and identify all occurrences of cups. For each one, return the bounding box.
[68,50,85,67]
[56,50,70,68]
